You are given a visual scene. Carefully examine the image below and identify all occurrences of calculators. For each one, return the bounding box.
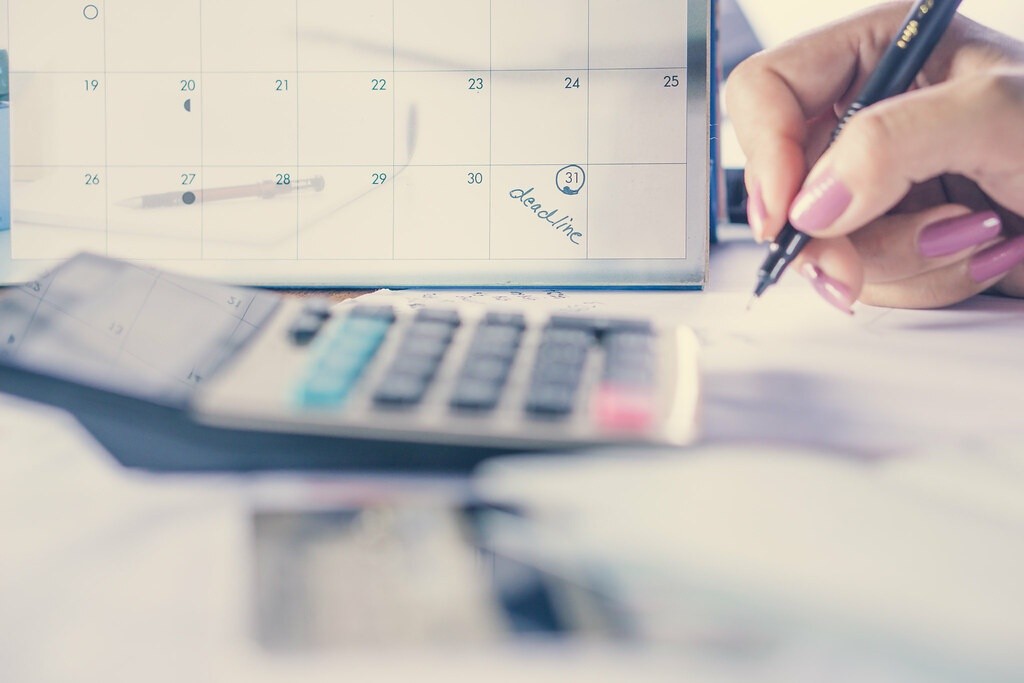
[0,250,702,474]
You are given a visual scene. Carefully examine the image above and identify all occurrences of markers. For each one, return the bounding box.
[753,0,962,297]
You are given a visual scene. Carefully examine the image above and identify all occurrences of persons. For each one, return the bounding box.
[722,2,1023,311]
